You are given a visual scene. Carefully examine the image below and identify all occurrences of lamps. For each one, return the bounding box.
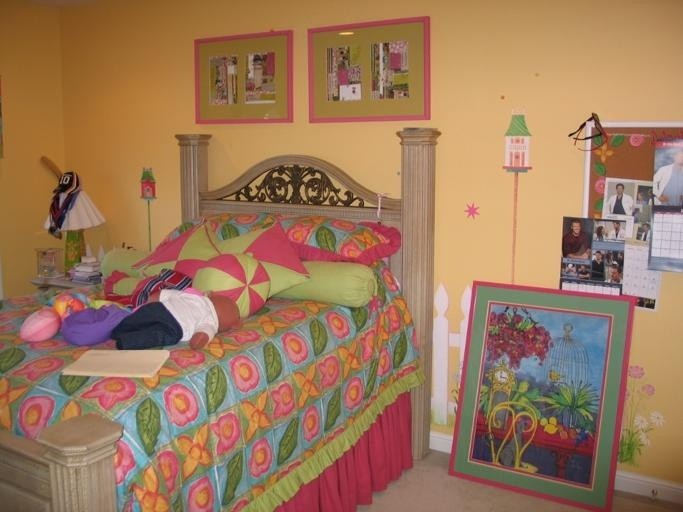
[45,189,107,272]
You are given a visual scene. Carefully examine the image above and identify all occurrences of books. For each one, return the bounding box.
[71,260,101,286]
[60,347,171,380]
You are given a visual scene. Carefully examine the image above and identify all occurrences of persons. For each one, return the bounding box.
[560,183,654,285]
[653,147,683,205]
[92,287,240,353]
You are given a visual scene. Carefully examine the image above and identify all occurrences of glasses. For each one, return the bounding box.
[574,127,608,151]
[568,113,603,140]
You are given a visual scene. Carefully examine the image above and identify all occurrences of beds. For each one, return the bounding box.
[2,129,449,510]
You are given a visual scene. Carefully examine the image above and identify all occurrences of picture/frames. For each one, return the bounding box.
[192,15,432,125]
[451,281,635,511]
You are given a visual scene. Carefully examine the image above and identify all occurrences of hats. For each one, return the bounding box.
[53,172,79,194]
